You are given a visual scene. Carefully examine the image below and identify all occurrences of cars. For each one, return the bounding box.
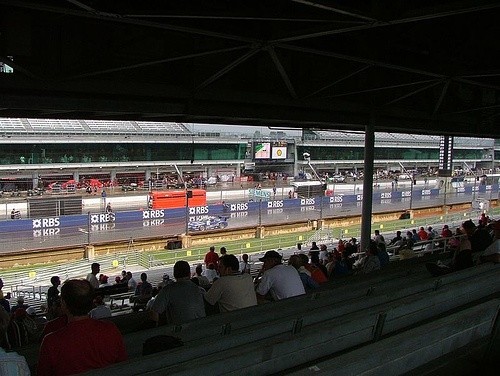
[394,173,412,180]
[328,176,344,183]
[28,188,46,193]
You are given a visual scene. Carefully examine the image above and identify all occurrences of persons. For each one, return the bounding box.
[479,201,484,211]
[0,251,212,376]
[0,177,214,192]
[273,185,277,196]
[203,212,499,322]
[222,201,229,212]
[288,190,294,200]
[11,208,16,220]
[240,168,500,189]
[106,202,113,213]
[19,152,110,166]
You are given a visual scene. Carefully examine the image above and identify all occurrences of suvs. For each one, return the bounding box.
[422,171,427,176]
[122,184,136,191]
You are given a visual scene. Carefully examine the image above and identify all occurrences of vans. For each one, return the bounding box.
[407,170,417,175]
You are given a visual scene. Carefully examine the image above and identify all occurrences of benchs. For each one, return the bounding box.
[0,211,500,376]
[1,164,499,203]
